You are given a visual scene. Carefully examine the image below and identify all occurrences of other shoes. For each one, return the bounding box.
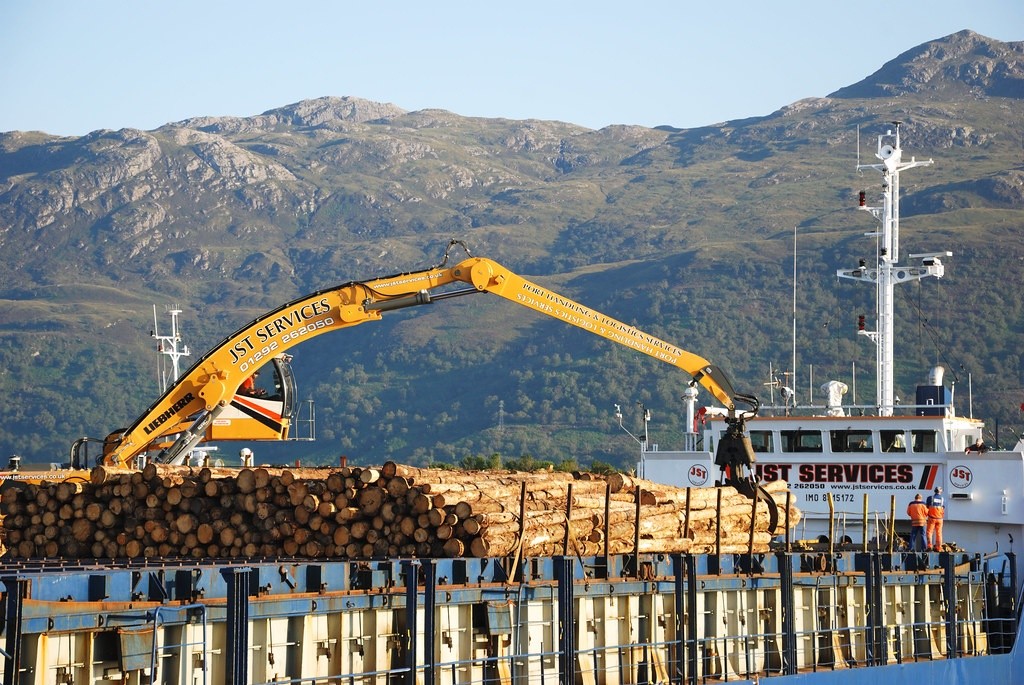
[934,549,945,552]
[906,549,914,552]
[925,547,931,552]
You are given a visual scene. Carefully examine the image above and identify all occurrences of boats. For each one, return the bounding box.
[615,122,1024,590]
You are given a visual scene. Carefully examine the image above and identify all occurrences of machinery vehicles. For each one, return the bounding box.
[0,237,758,489]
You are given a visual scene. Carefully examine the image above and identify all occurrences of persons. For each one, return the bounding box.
[965,438,989,455]
[243,371,266,394]
[907,493,929,552]
[925,487,945,552]
